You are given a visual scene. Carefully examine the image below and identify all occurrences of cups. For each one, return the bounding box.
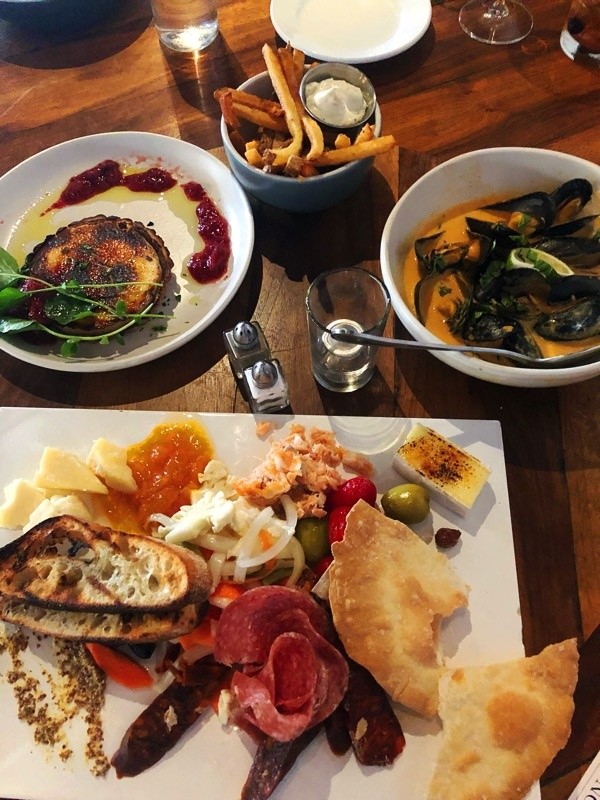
[299,63,377,128]
[303,267,391,392]
[559,1,600,67]
[148,0,219,52]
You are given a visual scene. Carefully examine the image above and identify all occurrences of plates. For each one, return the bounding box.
[0,405,541,800]
[0,131,254,373]
[269,1,433,65]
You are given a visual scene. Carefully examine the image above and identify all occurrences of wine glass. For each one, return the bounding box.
[457,1,534,45]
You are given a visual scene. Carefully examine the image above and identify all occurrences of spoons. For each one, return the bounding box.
[329,327,600,367]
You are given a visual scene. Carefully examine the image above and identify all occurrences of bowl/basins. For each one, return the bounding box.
[218,65,382,211]
[378,147,600,388]
